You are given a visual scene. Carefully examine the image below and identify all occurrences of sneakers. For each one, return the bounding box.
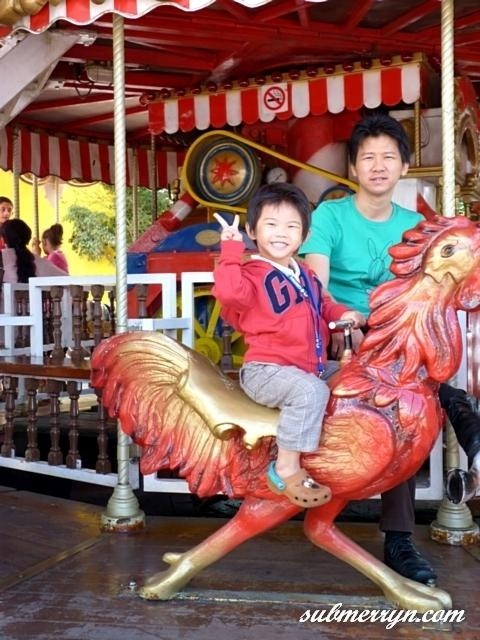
[445,388,480,457]
[384,537,437,586]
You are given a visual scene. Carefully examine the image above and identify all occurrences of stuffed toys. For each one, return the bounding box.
[91,215,480,615]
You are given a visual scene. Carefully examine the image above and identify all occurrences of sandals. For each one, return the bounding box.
[268,461,331,508]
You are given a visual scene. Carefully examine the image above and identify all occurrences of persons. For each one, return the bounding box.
[0,196,14,249]
[213,183,366,508]
[32,223,69,273]
[296,114,480,587]
[0,220,71,283]
